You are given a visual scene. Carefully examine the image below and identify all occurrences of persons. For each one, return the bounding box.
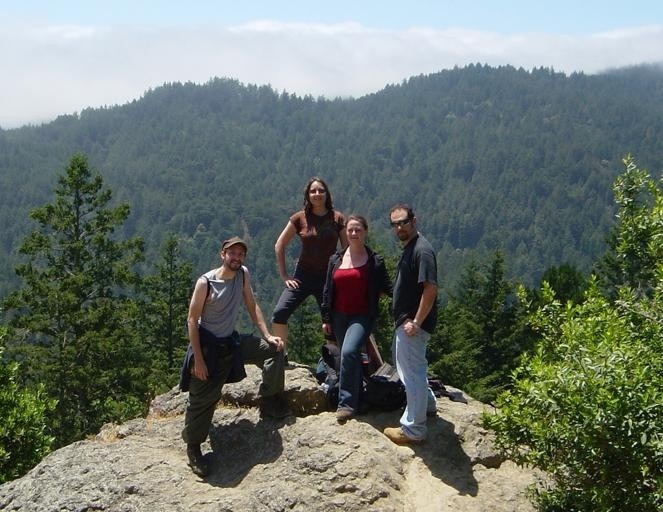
[320,214,395,421]
[271,178,348,364]
[384,204,438,444]
[182,236,293,477]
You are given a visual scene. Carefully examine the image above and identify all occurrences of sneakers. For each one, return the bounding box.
[385,426,423,447]
[337,407,354,420]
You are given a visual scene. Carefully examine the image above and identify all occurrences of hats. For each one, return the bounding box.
[221,235,247,251]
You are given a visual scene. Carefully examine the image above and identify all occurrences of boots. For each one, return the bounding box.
[260,395,291,418]
[186,442,209,476]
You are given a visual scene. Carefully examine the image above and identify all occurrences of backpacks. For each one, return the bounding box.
[316,342,450,413]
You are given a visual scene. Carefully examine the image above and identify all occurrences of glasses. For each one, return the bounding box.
[390,220,409,227]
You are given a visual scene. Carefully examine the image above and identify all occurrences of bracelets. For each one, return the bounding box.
[413,321,421,327]
